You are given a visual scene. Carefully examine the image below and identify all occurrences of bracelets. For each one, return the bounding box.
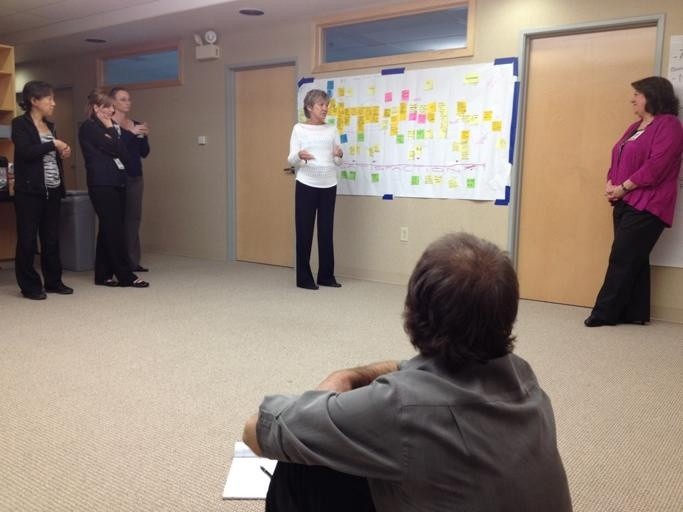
[620,182,630,193]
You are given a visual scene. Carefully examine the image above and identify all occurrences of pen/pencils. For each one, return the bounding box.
[261,467,272,478]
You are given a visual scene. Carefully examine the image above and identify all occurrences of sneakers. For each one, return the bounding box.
[582,315,652,329]
[24,284,74,301]
[294,279,345,292]
[95,264,152,290]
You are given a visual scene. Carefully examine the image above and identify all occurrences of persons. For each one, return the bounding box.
[11,81,73,299]
[242,231,571,511]
[286,89,342,290]
[76,88,150,288]
[107,87,150,272]
[581,76,680,327]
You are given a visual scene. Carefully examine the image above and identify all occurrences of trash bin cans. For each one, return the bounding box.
[59,191,95,271]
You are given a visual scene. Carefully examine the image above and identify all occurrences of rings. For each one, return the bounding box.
[64,146,67,150]
